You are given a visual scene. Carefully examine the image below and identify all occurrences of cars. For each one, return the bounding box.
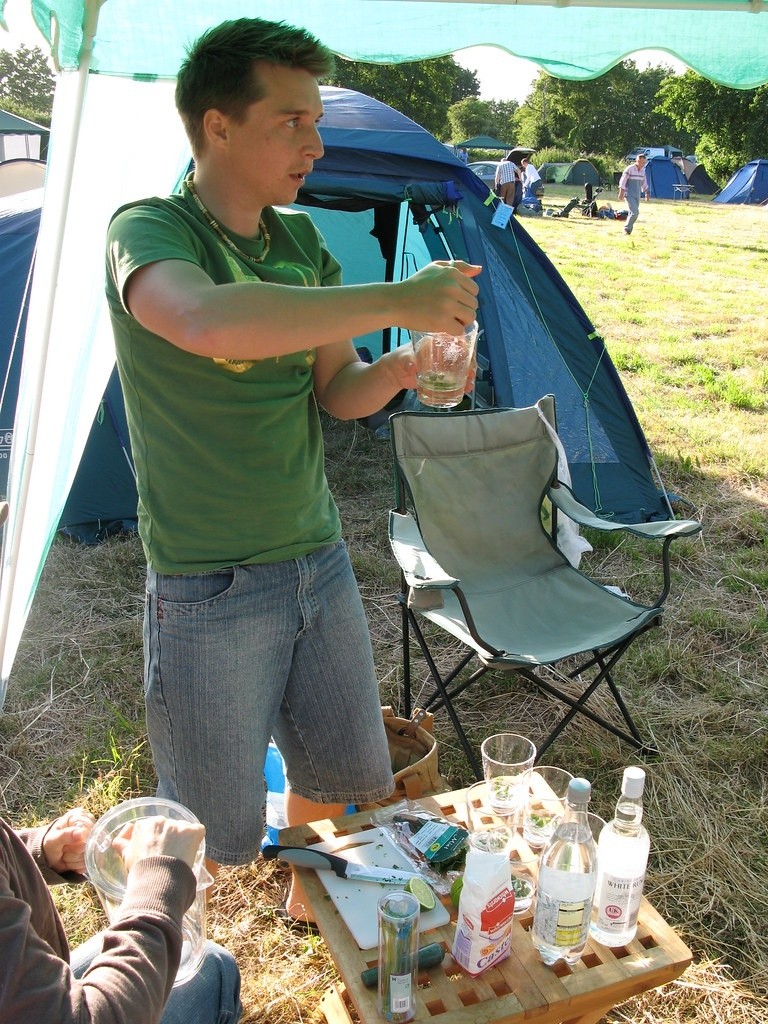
[465,148,545,198]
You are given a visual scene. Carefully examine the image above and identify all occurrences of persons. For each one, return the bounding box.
[0,806,243,1024]
[618,153,650,235]
[521,158,542,197]
[494,157,521,206]
[458,147,469,165]
[106,17,482,907]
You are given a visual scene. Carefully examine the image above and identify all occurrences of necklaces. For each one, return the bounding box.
[186,170,270,264]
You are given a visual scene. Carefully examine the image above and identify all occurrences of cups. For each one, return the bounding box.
[409,319,479,409]
[520,765,574,848]
[481,733,537,812]
[551,810,608,844]
[69,796,213,990]
[463,779,522,843]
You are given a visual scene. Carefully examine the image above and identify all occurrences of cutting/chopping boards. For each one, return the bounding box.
[306,828,451,949]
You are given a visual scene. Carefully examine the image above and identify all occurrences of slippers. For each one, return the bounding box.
[285,917,319,934]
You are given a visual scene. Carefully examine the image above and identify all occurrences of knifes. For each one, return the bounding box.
[262,845,438,886]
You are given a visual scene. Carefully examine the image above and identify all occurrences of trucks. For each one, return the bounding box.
[626,146,684,162]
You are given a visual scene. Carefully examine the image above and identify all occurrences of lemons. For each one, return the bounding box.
[404,877,436,912]
[451,876,463,910]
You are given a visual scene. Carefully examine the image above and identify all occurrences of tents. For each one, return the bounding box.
[670,156,723,195]
[0,82,679,546]
[640,155,690,199]
[657,144,682,158]
[454,135,516,160]
[710,158,768,206]
[536,158,605,186]
[0,0,768,716]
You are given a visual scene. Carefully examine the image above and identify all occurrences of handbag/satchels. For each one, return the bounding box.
[534,405,594,570]
[355,705,453,811]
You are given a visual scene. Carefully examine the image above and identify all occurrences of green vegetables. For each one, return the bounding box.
[511,874,531,898]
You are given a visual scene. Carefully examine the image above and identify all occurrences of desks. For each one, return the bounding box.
[673,185,695,201]
[278,770,695,1024]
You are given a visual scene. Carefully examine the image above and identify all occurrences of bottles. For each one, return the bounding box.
[589,766,651,948]
[529,777,599,967]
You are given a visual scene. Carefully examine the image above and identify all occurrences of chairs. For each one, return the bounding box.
[386,396,705,784]
[572,187,605,219]
